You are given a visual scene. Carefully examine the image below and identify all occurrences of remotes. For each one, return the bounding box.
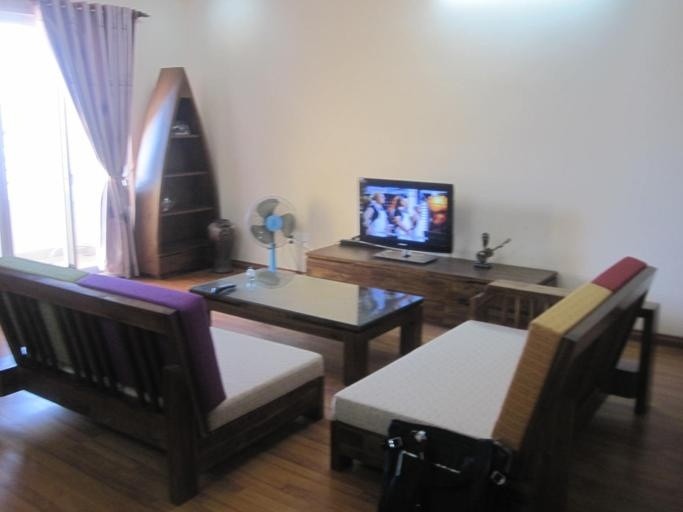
[211,283,236,295]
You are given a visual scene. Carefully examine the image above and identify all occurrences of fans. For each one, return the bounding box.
[243,194,298,287]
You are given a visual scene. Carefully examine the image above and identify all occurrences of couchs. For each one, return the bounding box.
[327,257,662,509]
[1,250,329,505]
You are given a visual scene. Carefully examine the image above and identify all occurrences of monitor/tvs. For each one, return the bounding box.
[360,177,452,264]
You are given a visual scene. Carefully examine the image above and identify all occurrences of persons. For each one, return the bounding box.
[358,285,416,320]
[387,193,433,244]
[361,191,391,237]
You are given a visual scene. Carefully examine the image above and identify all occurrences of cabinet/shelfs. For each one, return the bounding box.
[306,242,558,332]
[134,67,220,279]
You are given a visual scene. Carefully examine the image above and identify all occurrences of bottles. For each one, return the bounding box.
[246,268,256,291]
